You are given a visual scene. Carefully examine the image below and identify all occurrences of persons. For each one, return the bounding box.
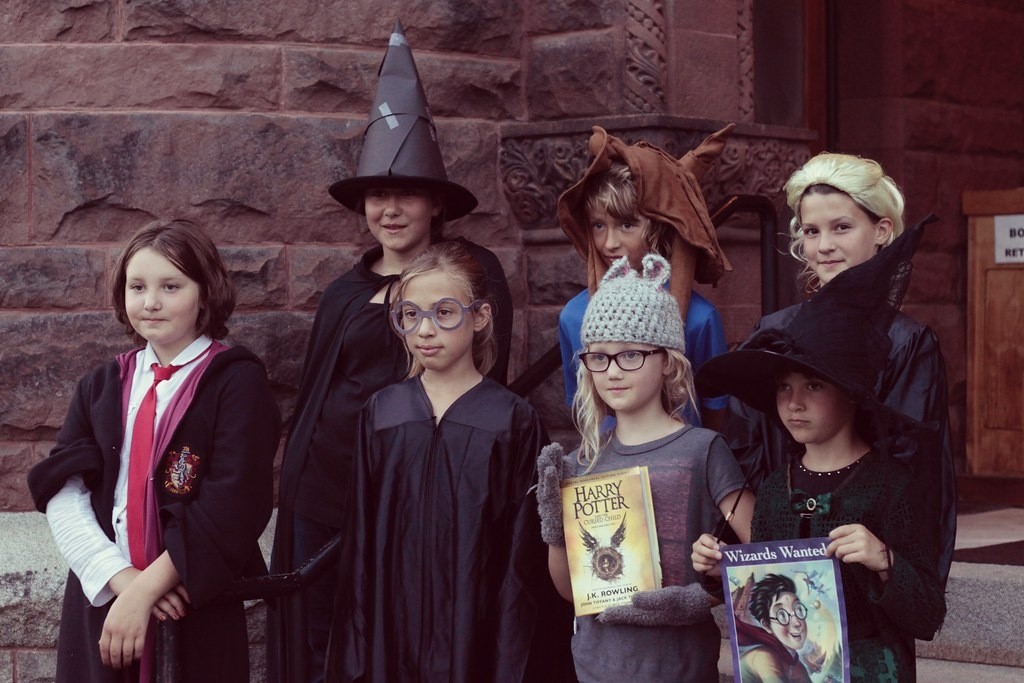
[535,253,755,683]
[324,239,554,682]
[722,151,957,589]
[27,216,284,683]
[264,181,517,683]
[555,119,731,442]
[696,212,949,683]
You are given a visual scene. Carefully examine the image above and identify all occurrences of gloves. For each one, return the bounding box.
[536,444,573,543]
[600,581,714,626]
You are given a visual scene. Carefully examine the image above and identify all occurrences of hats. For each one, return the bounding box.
[327,16,476,223]
[694,211,941,418]
[580,255,686,354]
[557,122,739,325]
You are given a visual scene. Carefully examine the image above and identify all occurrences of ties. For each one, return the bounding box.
[128,342,216,572]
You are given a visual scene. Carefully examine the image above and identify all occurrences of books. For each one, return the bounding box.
[559,465,663,618]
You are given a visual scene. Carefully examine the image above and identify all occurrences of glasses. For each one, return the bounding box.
[389,298,481,335]
[578,348,665,372]
